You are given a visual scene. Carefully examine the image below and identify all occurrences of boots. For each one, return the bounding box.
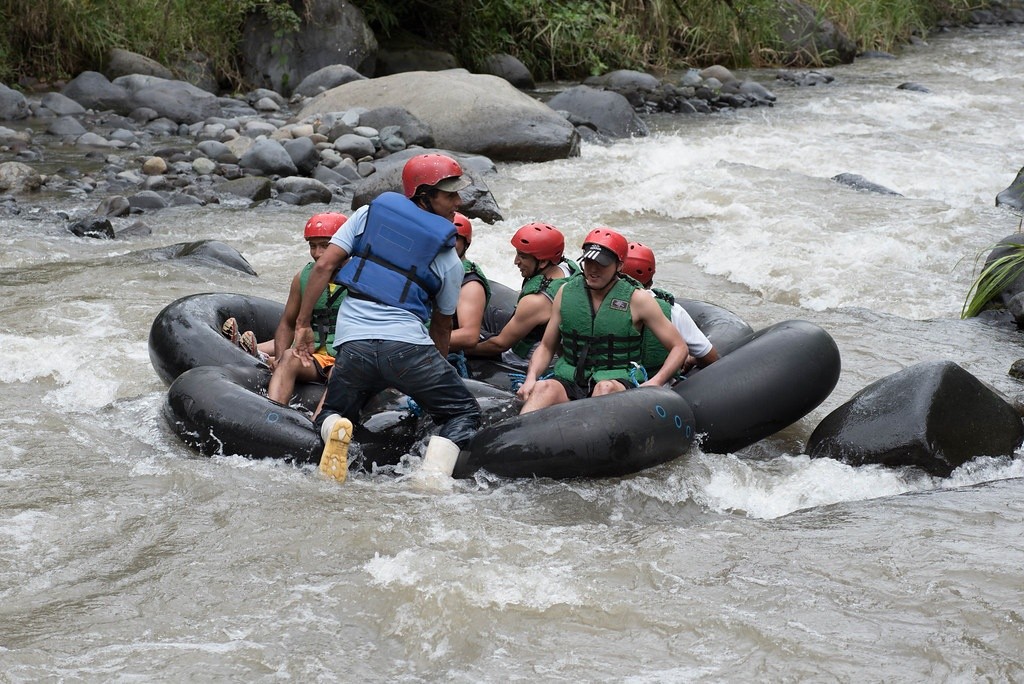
[422,435,461,479]
[319,413,353,486]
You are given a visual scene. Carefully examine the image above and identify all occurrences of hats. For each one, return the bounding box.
[577,243,618,267]
[437,177,471,193]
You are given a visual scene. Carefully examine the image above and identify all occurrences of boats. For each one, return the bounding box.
[147,278,841,482]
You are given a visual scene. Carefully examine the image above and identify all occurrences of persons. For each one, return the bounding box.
[291,154,483,487]
[267,213,350,421]
[518,227,690,416]
[620,242,719,387]
[461,222,582,375]
[221,213,493,363]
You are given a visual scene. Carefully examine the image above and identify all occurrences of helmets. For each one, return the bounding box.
[622,242,656,285]
[510,222,564,265]
[452,211,472,245]
[304,211,348,241]
[582,228,628,262]
[402,154,472,199]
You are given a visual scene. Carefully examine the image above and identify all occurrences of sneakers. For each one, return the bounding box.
[222,317,241,346]
[240,331,270,365]
